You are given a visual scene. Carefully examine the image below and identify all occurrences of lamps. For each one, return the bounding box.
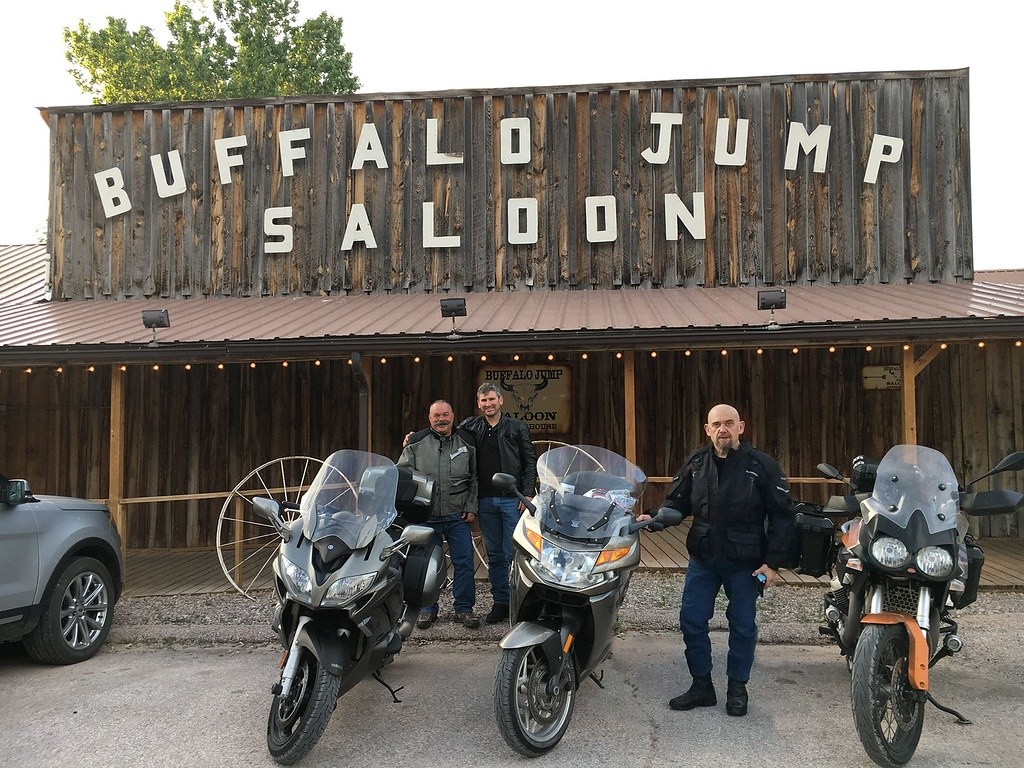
[757,289,786,330]
[441,298,467,339]
[142,309,171,348]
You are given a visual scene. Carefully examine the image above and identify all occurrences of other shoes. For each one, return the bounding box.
[726,683,749,716]
[416,611,438,629]
[669,677,717,711]
[454,610,480,627]
[486,603,509,624]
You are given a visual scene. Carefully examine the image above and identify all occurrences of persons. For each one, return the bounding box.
[635,404,797,717]
[394,400,480,629]
[401,383,538,625]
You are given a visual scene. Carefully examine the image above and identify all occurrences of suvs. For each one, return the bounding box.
[1,475,126,666]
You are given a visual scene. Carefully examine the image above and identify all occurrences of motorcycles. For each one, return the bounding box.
[252,449,448,765]
[817,443,1023,768]
[491,439,685,759]
